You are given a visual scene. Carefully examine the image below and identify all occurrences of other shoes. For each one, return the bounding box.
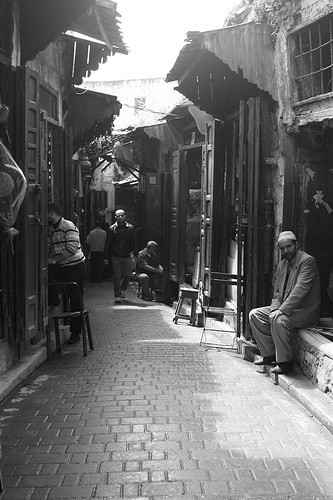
[144,296,151,301]
[114,297,121,302]
[121,289,125,298]
[48,297,61,306]
[270,363,293,373]
[254,357,275,365]
[67,334,80,345]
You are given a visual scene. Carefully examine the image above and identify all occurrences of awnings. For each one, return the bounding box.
[120,122,176,147]
[165,19,278,124]
[20,0,128,88]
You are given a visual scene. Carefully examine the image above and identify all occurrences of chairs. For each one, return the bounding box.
[48,281,95,358]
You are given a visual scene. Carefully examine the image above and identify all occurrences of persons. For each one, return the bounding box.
[47,203,87,345]
[104,209,139,302]
[87,223,108,281]
[248,231,322,374]
[136,241,164,300]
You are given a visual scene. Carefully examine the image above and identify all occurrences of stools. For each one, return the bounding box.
[137,277,155,299]
[172,287,200,326]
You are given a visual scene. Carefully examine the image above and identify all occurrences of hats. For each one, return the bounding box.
[277,231,297,247]
